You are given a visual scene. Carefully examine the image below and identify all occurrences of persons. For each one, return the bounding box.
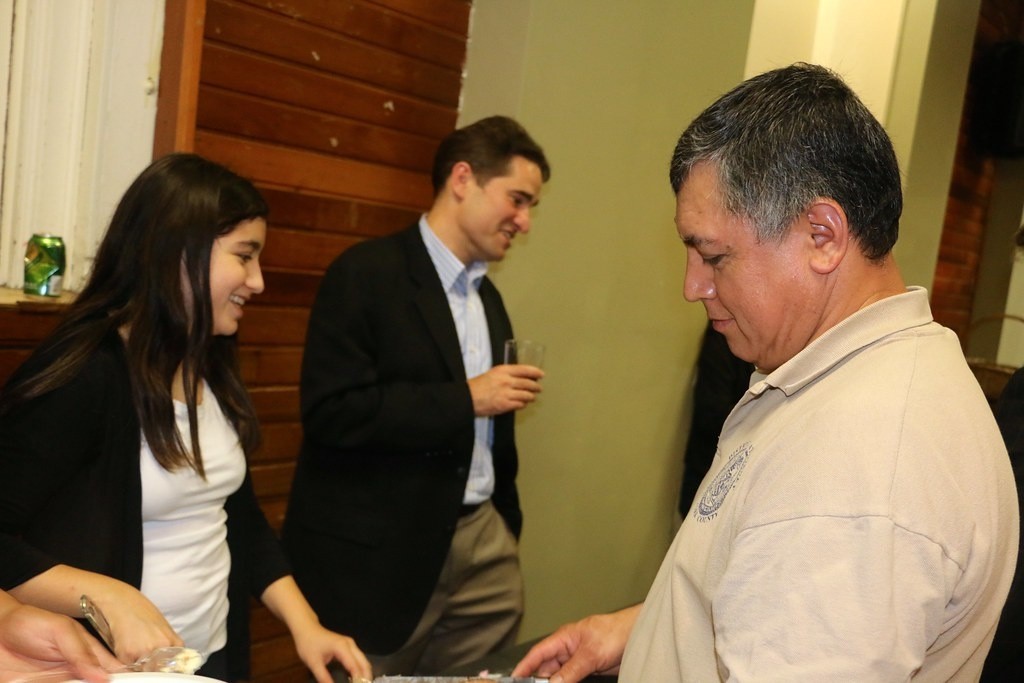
[980,229,1024,682]
[0,587,130,683]
[277,115,551,683]
[510,63,1020,683]
[0,152,373,683]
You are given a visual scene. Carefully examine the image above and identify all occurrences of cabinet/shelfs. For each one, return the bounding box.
[0,286,78,388]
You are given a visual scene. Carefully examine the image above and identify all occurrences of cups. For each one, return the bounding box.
[503,338,546,368]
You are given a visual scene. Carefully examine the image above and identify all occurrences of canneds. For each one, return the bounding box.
[23,232,65,299]
